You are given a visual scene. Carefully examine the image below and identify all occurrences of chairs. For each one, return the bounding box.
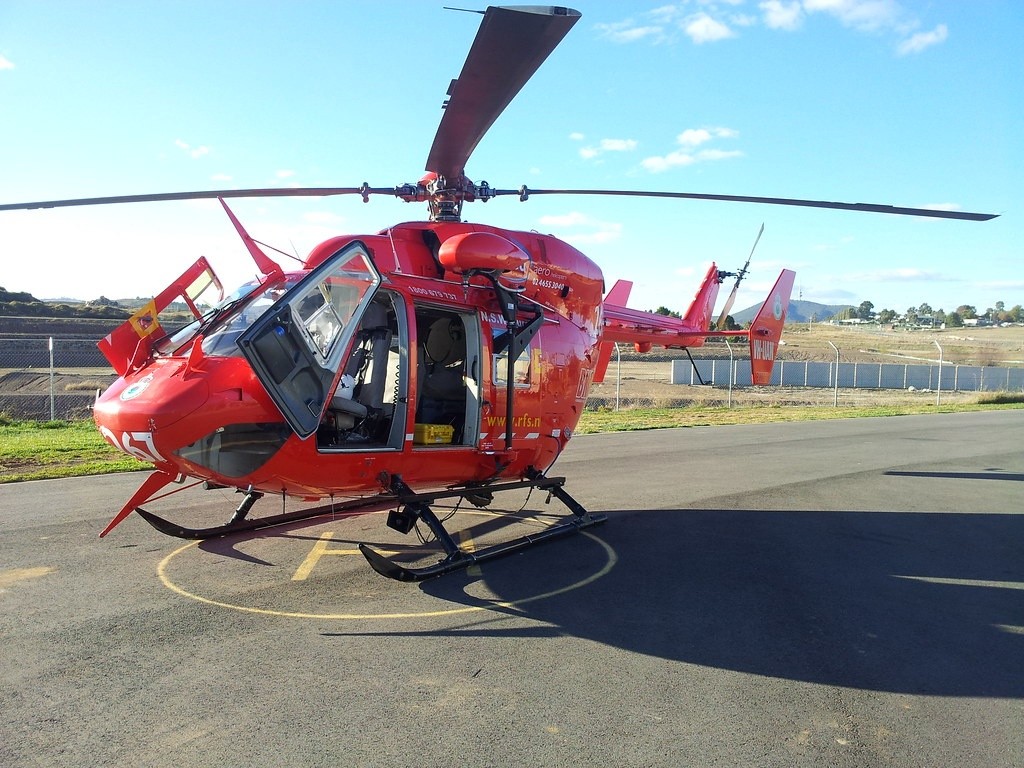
[328,303,393,445]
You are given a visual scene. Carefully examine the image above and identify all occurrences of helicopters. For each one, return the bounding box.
[0,1,1003,585]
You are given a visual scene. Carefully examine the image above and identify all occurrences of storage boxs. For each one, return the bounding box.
[414,424,455,445]
